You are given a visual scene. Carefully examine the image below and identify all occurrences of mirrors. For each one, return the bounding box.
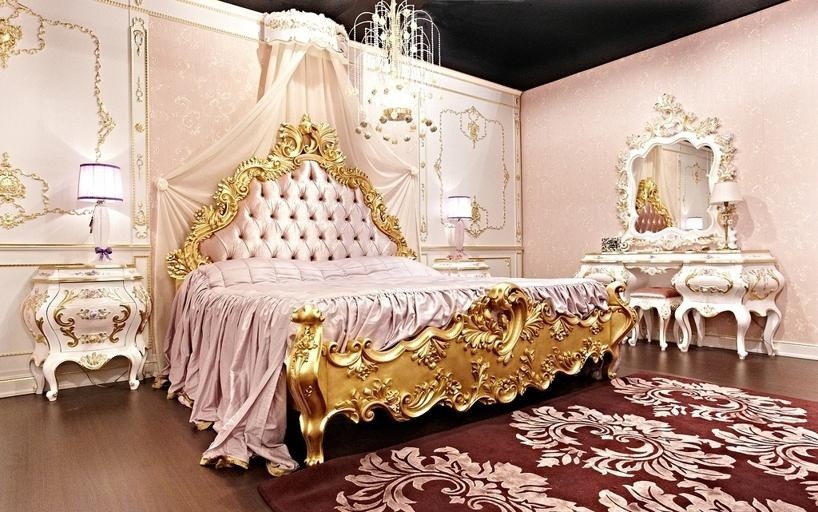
[615,91,738,251]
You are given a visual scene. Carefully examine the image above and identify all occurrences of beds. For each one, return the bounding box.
[151,113,640,476]
[635,178,673,234]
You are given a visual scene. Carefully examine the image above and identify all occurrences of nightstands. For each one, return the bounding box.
[431,260,491,277]
[21,267,152,401]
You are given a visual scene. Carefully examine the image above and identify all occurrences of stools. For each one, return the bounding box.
[628,286,692,352]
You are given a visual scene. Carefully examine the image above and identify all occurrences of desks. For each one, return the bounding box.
[575,250,785,360]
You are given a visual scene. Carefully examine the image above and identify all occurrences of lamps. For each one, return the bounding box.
[445,195,474,261]
[78,164,124,268]
[683,217,703,230]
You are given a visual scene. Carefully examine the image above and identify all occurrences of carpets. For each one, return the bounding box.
[258,367,818,512]
